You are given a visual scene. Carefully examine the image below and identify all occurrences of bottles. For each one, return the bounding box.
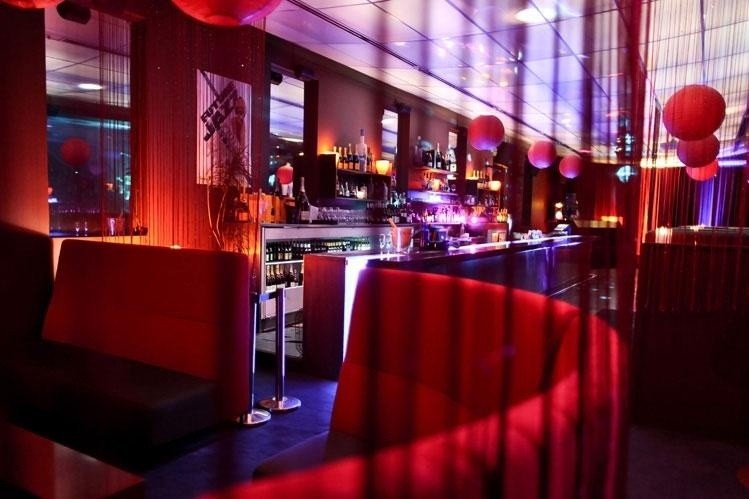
[266,237,373,292]
[460,224,465,236]
[420,225,430,249]
[296,175,310,222]
[374,234,391,249]
[333,128,509,224]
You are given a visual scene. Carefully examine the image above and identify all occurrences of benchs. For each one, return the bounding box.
[30,237,255,438]
[194,264,631,498]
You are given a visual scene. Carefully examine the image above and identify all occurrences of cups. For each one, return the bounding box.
[106,217,126,236]
[391,227,413,248]
[487,230,506,242]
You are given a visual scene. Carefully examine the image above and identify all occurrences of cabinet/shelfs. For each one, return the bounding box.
[413,164,460,196]
[318,152,396,207]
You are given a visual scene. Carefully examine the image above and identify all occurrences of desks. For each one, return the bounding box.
[0,411,146,497]
